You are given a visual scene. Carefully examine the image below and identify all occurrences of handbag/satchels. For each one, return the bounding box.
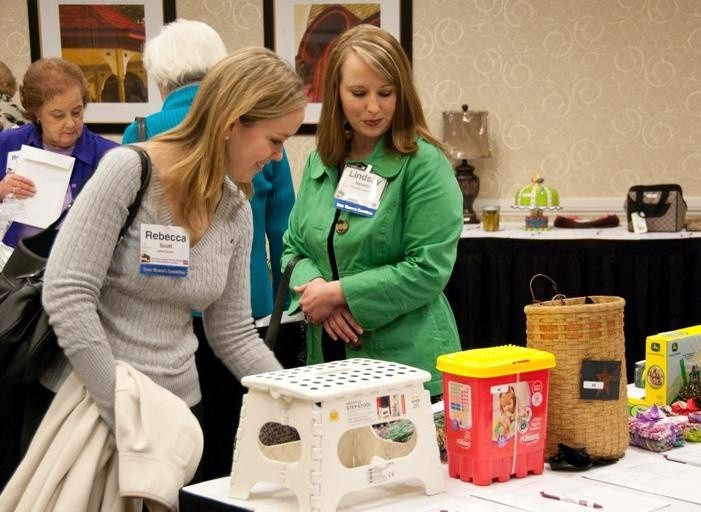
[625,183,688,233]
[0,143,153,388]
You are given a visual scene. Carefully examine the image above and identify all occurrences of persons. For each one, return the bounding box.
[0,56,120,498]
[281,23,465,404]
[0,45,309,512]
[121,17,296,512]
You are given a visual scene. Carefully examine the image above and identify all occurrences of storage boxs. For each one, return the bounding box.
[435,340,561,487]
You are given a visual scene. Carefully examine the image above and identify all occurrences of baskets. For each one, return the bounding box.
[522,272,630,463]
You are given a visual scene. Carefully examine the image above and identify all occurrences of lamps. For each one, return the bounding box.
[440,101,492,225]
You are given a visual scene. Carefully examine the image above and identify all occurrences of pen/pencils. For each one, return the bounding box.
[541,491,603,508]
[663,454,701,467]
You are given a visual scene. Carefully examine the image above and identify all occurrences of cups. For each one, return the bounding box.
[482,206,501,232]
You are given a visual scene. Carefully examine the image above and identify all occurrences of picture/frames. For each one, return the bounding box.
[24,1,180,139]
[261,1,416,137]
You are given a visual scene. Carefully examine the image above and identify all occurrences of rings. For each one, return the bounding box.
[11,185,18,194]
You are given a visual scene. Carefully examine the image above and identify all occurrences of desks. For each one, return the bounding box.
[176,374,701,512]
[445,216,701,390]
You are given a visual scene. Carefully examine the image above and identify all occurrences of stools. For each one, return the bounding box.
[226,356,448,512]
